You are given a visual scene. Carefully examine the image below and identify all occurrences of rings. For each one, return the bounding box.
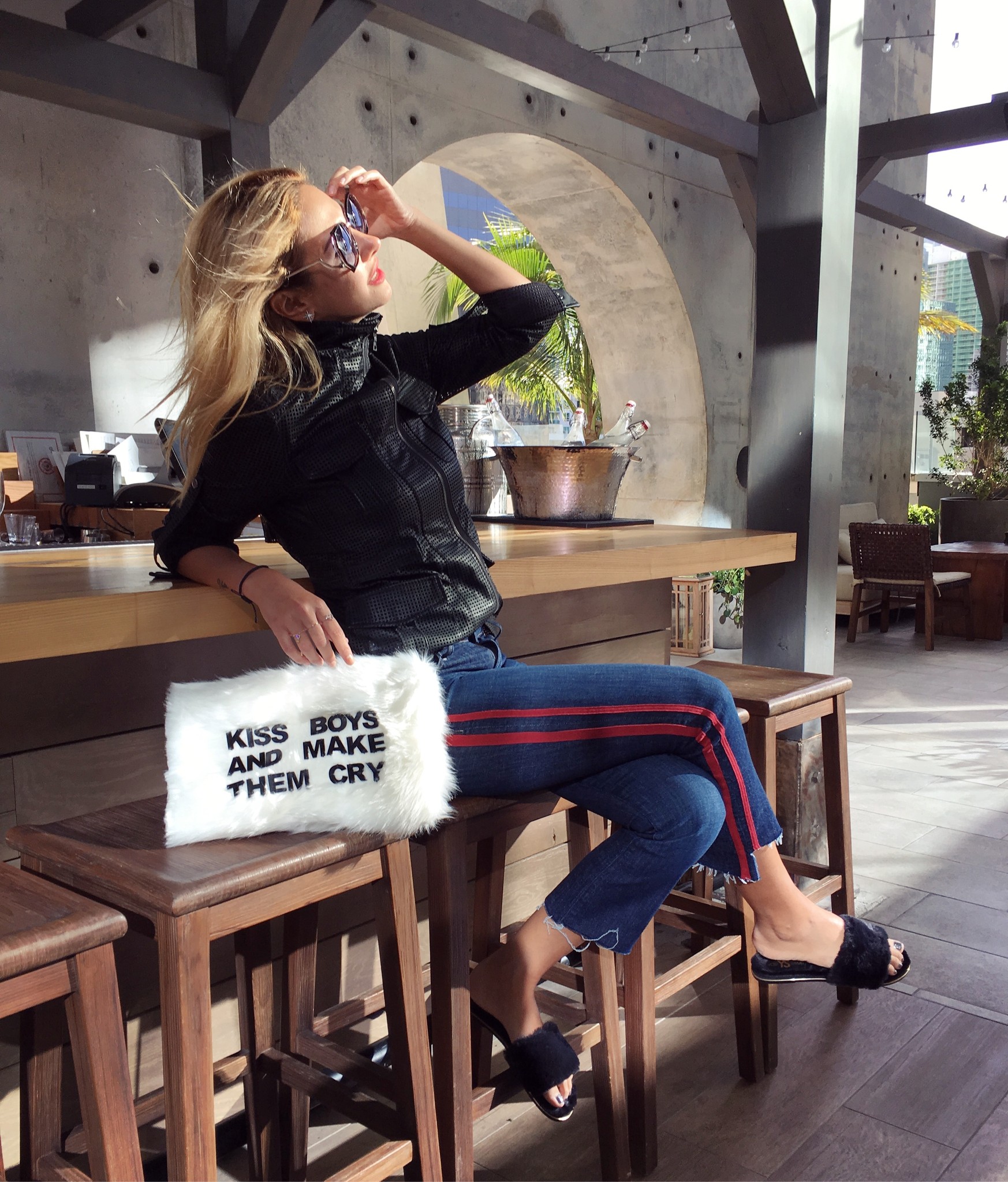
[293,629,307,642]
[306,622,320,631]
[319,614,335,626]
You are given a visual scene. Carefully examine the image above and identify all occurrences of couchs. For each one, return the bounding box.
[835,502,915,633]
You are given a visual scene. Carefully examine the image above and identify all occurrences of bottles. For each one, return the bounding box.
[561,408,587,447]
[587,419,651,446]
[485,394,524,447]
[604,401,636,437]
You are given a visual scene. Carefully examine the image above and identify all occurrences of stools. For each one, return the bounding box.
[0,659,858,1182]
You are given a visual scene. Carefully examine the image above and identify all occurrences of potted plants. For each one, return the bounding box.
[918,320,1008,545]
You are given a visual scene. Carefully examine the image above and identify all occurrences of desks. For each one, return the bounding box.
[915,541,1008,641]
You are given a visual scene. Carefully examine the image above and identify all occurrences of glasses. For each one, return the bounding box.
[286,184,369,279]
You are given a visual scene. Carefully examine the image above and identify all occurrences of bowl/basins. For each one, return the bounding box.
[489,446,643,523]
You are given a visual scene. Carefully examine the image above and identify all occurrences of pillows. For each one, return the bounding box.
[838,529,852,564]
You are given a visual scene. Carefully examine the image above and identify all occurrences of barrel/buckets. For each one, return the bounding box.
[438,404,502,516]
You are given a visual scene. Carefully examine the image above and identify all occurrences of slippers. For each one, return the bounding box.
[751,915,911,990]
[470,998,581,1121]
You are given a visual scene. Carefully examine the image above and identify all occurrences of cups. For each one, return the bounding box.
[4,514,36,545]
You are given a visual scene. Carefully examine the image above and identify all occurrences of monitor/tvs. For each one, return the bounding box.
[155,418,188,485]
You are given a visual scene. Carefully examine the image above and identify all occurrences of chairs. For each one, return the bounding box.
[849,523,973,652]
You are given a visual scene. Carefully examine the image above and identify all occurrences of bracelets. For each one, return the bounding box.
[152,168,912,1128]
[230,562,275,624]
